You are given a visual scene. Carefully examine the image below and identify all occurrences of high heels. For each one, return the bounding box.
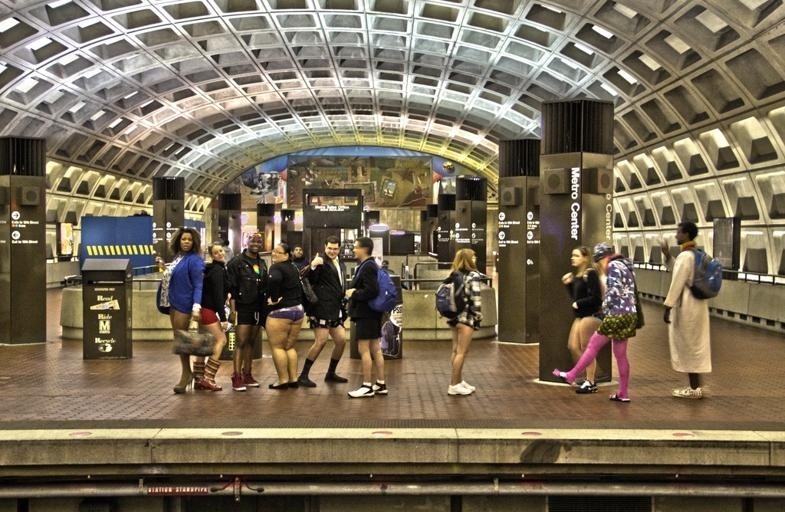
[172,373,193,393]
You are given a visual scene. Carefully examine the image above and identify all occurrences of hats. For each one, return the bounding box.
[593,242,615,262]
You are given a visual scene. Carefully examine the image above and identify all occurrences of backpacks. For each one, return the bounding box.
[584,268,607,316]
[434,270,470,319]
[684,246,723,300]
[357,260,399,314]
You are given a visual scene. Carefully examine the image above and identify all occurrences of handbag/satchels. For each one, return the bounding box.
[291,262,318,304]
[170,319,217,357]
[635,283,644,330]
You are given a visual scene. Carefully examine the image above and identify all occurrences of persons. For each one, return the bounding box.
[662,222,712,399]
[226,231,268,392]
[155,226,206,392]
[194,241,229,391]
[223,240,234,268]
[348,237,388,397]
[263,243,304,389]
[296,236,348,386]
[381,260,394,274]
[562,245,605,392]
[290,244,309,275]
[553,241,640,403]
[445,248,482,395]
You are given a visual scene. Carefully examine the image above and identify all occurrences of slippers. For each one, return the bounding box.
[553,368,573,385]
[608,393,630,402]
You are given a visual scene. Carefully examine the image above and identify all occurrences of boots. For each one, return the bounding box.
[201,358,222,391]
[192,360,205,389]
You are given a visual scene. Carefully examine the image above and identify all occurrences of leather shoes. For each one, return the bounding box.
[269,373,348,390]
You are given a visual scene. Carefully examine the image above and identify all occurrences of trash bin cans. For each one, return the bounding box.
[81,258,133,360]
[350,275,403,359]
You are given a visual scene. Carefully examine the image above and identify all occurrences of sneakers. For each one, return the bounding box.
[231,371,247,391]
[242,368,259,387]
[447,382,472,395]
[461,380,476,393]
[347,384,375,398]
[575,379,598,394]
[373,381,388,395]
[697,388,702,399]
[671,386,698,399]
